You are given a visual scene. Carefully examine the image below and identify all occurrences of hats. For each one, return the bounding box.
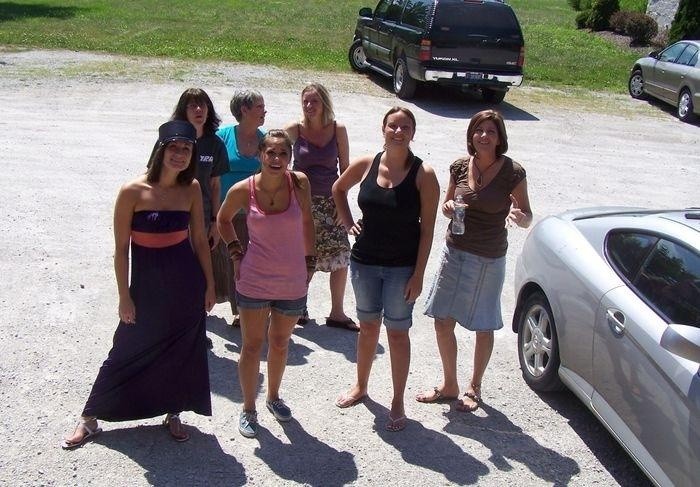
[158,121,197,142]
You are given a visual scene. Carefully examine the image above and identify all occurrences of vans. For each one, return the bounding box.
[347,0,526,105]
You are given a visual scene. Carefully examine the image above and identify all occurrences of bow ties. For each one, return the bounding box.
[265,398,291,421]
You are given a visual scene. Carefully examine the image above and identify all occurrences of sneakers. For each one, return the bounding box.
[233,315,240,327]
[239,409,258,438]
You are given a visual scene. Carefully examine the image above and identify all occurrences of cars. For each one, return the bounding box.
[512,203,700,487]
[628,38,700,121]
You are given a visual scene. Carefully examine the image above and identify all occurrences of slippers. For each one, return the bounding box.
[327,315,360,331]
[417,386,459,402]
[456,389,483,412]
[386,414,406,431]
[336,391,369,408]
[295,308,308,324]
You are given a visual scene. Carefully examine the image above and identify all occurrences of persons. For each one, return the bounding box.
[332,106,440,431]
[59,122,213,449]
[416,110,532,413]
[216,129,316,437]
[282,84,361,332]
[146,88,228,350]
[217,91,270,328]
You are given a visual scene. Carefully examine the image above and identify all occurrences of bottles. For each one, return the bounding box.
[452,194,465,235]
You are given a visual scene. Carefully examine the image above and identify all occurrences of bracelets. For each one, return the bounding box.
[305,255,317,273]
[225,238,244,262]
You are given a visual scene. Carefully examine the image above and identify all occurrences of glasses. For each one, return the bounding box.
[186,103,206,108]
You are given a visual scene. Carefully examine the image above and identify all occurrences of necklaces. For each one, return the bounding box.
[153,181,175,203]
[264,185,279,206]
[473,156,498,187]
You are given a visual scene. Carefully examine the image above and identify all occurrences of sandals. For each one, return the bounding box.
[163,413,190,442]
[61,419,102,449]
